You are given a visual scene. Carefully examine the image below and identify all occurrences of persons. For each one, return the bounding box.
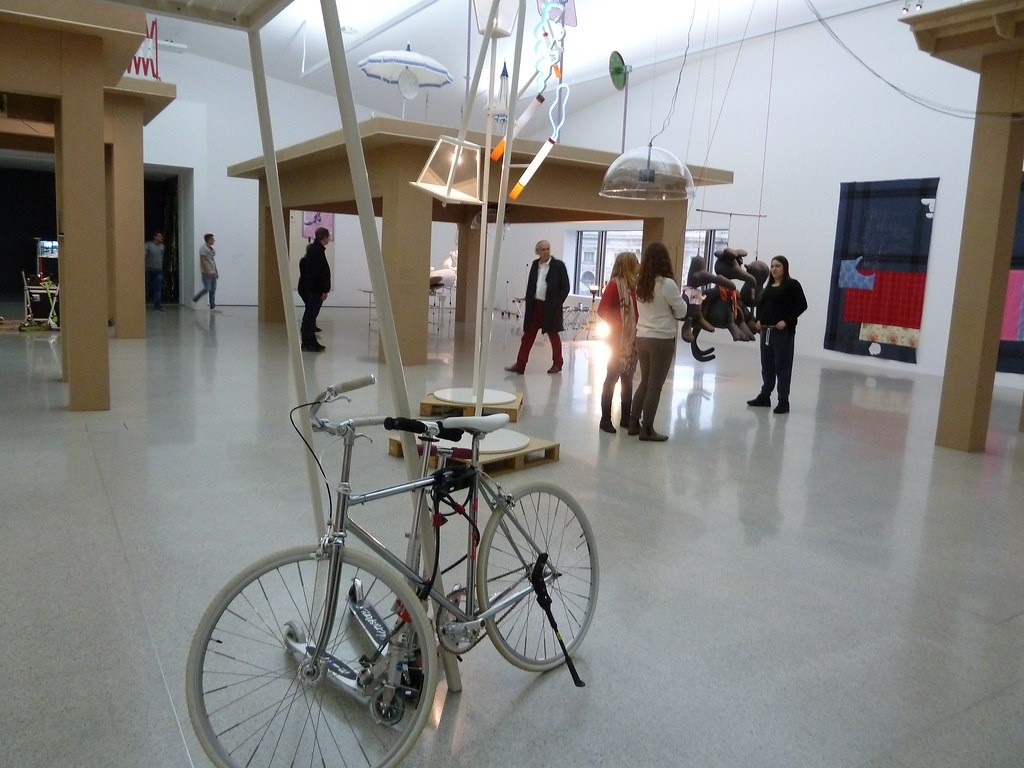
[627,243,688,441]
[193,234,223,313]
[597,252,640,433]
[504,240,570,376]
[144,231,166,312]
[299,228,331,354]
[746,256,808,414]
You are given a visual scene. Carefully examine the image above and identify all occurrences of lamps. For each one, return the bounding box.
[408,0,695,447]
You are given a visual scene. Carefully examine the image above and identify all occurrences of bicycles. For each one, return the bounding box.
[185,371,601,768]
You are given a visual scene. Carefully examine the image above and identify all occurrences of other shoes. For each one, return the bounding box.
[153,304,165,311]
[504,366,525,374]
[746,396,771,406]
[547,365,562,373]
[209,306,221,312]
[773,401,790,413]
[300,325,321,333]
[189,299,199,310]
[301,340,325,352]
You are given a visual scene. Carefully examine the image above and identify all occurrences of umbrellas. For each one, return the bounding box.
[493,61,514,136]
[357,42,454,120]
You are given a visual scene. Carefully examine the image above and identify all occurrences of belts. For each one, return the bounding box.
[761,324,776,328]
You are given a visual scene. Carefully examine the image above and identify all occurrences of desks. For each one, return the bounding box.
[228,114,736,373]
[0,0,151,416]
[0,78,186,341]
[897,0,1024,457]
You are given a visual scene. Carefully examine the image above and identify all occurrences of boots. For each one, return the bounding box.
[620,401,631,427]
[627,399,641,435]
[599,404,617,433]
[638,406,669,440]
